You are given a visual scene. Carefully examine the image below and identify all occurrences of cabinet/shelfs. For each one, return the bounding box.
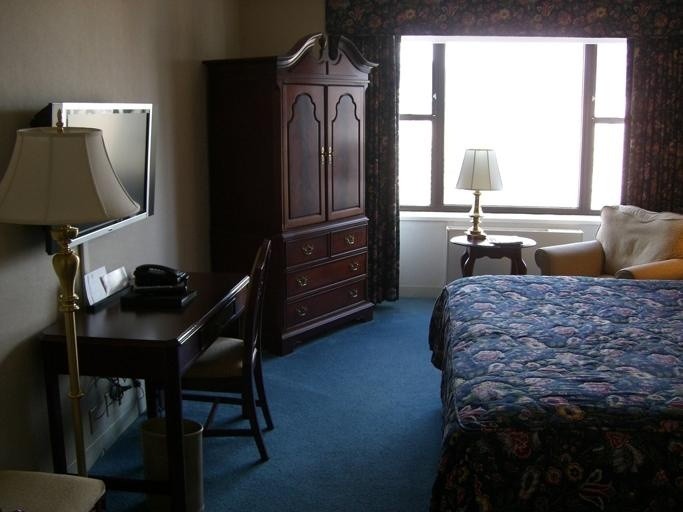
[202,32,381,357]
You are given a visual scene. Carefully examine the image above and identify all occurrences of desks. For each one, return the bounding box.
[37,268,252,511]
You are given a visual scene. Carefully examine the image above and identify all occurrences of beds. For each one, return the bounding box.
[428,274,683,511]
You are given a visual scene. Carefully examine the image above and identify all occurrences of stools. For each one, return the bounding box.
[0,469,108,511]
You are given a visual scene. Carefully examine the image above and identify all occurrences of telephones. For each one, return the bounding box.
[133,264,187,286]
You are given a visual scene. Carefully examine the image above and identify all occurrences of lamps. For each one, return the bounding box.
[455,148,504,240]
[0,109,141,477]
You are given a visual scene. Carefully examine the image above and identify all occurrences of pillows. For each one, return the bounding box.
[595,206,683,276]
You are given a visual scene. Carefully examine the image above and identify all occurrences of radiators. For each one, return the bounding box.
[446,225,584,285]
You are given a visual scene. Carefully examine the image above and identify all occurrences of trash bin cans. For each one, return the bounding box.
[140,417,204,512]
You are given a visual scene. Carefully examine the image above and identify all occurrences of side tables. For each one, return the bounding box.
[450,234,537,276]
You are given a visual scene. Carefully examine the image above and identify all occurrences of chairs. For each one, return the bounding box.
[535,205,683,280]
[145,238,274,460]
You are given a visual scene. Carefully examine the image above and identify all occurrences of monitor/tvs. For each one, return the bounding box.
[30,103,153,255]
